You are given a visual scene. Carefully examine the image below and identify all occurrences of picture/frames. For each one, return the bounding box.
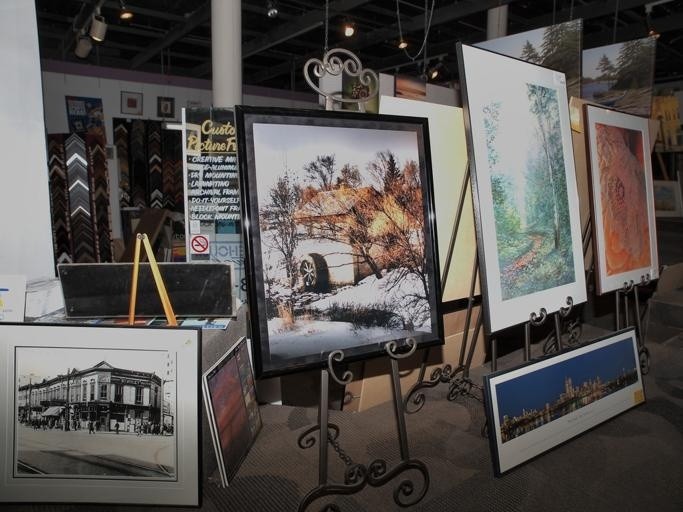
[203,337,267,489]
[582,103,660,298]
[41,41,211,257]
[457,44,589,333]
[308,14,683,218]
[1,322,200,505]
[234,105,445,377]
[484,328,646,478]
[58,263,233,320]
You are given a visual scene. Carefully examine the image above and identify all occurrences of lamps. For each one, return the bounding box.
[74,0,134,62]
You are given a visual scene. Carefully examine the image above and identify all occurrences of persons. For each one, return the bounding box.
[18,412,173,438]
